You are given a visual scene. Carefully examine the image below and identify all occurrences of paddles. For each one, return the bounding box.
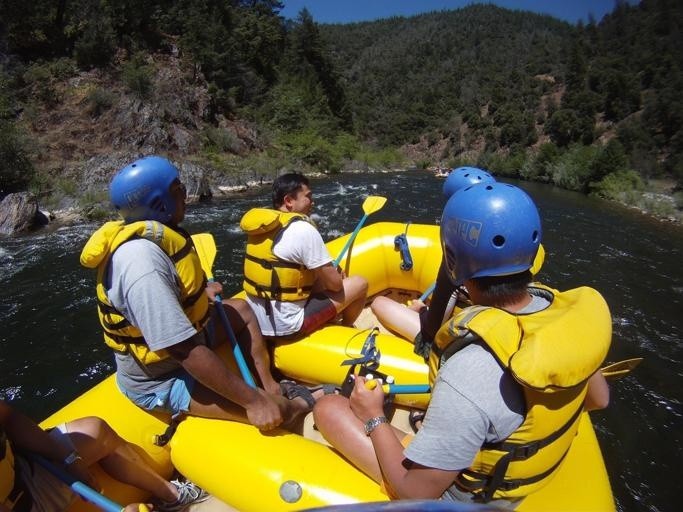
[332,196,388,270]
[190,233,258,388]
[364,358,644,393]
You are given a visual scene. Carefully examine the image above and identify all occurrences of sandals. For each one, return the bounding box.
[409,408,428,435]
[279,380,344,411]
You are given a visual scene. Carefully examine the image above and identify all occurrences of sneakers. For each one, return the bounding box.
[160,478,213,512]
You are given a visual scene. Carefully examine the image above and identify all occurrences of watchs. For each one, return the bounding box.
[360,415,390,434]
[62,445,82,468]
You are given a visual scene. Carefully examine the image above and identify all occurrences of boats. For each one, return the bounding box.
[35,222,617,511]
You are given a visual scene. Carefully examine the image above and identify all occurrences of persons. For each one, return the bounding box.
[315,181,612,509]
[0,400,211,512]
[80,157,342,431]
[370,166,497,361]
[240,173,366,342]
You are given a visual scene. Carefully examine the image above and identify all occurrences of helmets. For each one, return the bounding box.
[442,166,496,199]
[107,154,181,225]
[437,180,546,282]
